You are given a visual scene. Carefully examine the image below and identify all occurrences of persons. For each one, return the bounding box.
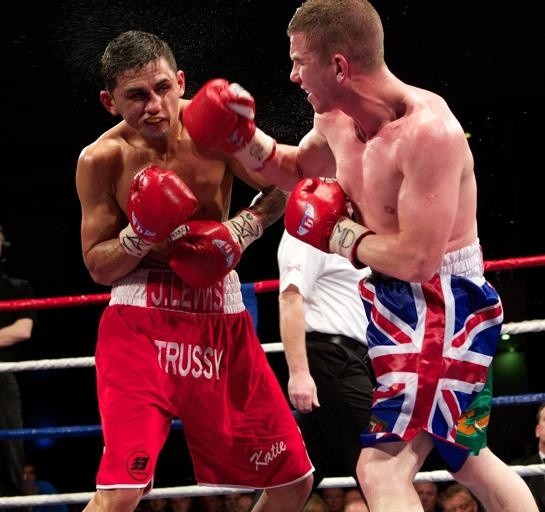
[1,464,67,511]
[1,0,315,512]
[1,235,36,496]
[152,481,477,512]
[279,230,372,504]
[181,0,542,512]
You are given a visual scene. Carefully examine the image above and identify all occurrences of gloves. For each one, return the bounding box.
[162,208,265,292]
[281,176,378,271]
[178,76,278,174]
[118,163,200,259]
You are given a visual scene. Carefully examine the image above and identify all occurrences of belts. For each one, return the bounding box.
[303,329,372,361]
